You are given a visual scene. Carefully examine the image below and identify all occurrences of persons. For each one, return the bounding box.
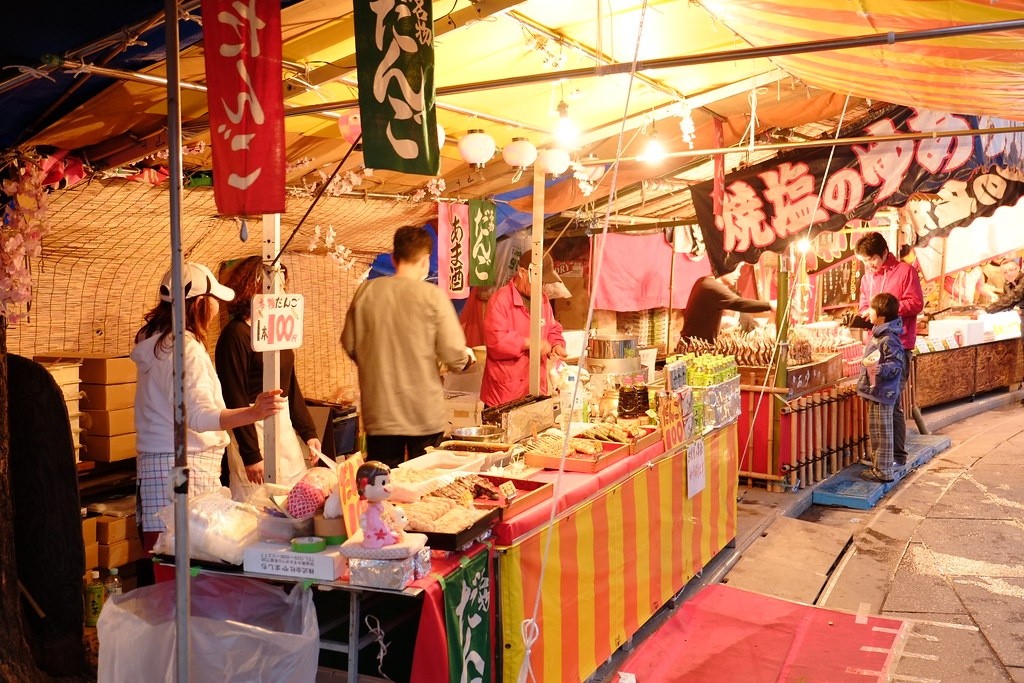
[676,264,777,349]
[214,256,322,490]
[129,263,283,589]
[8,353,97,682]
[480,250,571,427]
[355,461,403,549]
[855,233,924,465]
[855,293,904,483]
[973,259,1024,342]
[339,226,475,469]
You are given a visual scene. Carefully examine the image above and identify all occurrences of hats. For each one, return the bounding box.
[519,249,562,283]
[160,262,235,302]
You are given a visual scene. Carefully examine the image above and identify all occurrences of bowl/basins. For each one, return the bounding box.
[449,426,506,445]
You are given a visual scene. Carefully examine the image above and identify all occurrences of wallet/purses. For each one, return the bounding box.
[850,314,874,329]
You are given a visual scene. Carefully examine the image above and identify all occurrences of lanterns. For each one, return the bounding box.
[502,138,537,171]
[541,147,570,178]
[579,153,605,184]
[459,129,495,168]
[337,107,364,151]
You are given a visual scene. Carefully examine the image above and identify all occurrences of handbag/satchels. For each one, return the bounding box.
[96,572,320,683]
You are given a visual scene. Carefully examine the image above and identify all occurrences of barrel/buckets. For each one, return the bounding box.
[586,334,642,373]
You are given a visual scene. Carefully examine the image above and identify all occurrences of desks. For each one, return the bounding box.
[150,311,1023,681]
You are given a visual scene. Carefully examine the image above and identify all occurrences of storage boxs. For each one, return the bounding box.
[254,512,313,545]
[243,538,349,581]
[28,349,150,573]
[332,412,358,456]
[439,345,488,440]
[293,397,332,470]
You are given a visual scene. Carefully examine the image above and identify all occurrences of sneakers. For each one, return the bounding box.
[861,469,894,482]
[860,456,906,466]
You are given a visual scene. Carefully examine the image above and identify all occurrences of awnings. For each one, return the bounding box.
[0,0,1024,228]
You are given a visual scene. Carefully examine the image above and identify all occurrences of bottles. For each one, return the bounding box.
[84,571,105,629]
[634,376,650,417]
[618,377,637,419]
[560,375,582,436]
[692,392,704,439]
[663,353,737,391]
[105,567,123,598]
